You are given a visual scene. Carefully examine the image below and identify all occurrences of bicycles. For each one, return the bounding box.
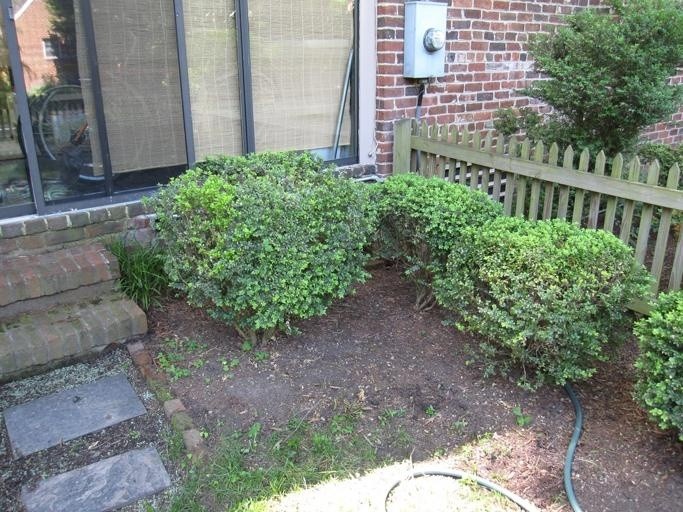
[16,21,285,187]
